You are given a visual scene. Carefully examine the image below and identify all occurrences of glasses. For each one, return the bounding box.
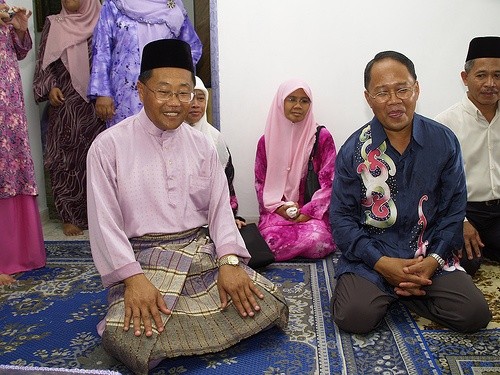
[285,98,312,104]
[368,82,416,103]
[140,81,196,102]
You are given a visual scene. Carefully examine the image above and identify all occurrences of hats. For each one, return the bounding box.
[465,36,500,62]
[140,39,194,73]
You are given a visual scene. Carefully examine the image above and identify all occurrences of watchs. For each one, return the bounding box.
[218,256,240,267]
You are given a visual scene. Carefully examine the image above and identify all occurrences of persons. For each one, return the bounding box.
[0,0,46,286]
[255,81,337,262]
[330,52,491,334]
[185,76,246,229]
[87,0,201,127]
[435,36,500,277]
[33,0,102,238]
[85,39,288,372]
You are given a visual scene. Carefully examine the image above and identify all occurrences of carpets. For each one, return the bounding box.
[0,241,500,375]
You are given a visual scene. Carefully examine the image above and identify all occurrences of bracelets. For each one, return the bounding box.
[428,254,445,270]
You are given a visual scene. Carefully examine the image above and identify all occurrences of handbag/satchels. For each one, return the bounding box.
[303,125,325,205]
